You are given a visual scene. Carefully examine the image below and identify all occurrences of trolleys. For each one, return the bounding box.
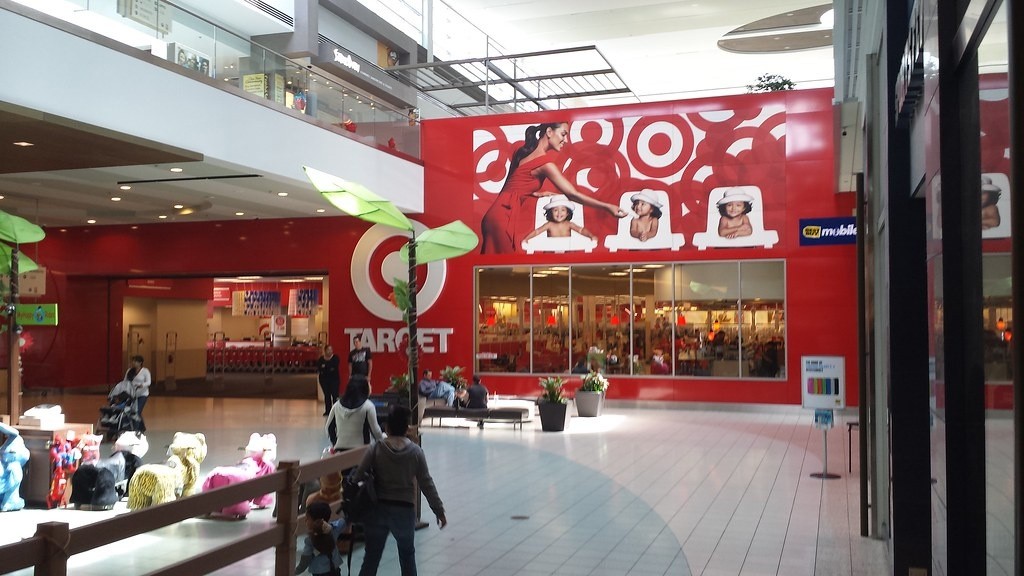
[207,340,322,374]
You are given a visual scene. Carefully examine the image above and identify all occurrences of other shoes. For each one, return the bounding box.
[477,423,484,429]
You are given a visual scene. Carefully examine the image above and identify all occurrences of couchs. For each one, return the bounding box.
[424,403,530,430]
[385,390,445,426]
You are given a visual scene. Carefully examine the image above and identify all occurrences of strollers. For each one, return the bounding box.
[94,379,140,443]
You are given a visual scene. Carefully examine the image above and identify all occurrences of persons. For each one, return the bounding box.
[463,376,489,429]
[430,378,462,407]
[178,49,209,75]
[296,447,346,576]
[982,177,1000,229]
[716,190,754,239]
[348,336,372,398]
[101,355,151,432]
[630,189,663,242]
[316,345,340,416]
[326,375,382,501]
[731,335,744,350]
[419,368,437,396]
[711,330,725,358]
[520,195,599,248]
[352,404,447,576]
[761,342,777,377]
[480,122,628,254]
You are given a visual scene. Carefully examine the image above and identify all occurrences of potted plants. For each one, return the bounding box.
[537,376,570,430]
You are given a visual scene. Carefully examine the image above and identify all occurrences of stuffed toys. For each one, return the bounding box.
[0,422,277,523]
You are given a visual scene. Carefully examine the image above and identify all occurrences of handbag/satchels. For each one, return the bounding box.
[347,440,380,505]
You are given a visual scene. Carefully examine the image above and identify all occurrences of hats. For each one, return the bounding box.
[543,194,576,212]
[630,189,664,209]
[717,188,754,205]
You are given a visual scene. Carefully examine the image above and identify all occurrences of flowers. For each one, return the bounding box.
[578,372,610,391]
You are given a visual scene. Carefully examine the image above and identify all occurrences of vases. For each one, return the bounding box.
[574,390,605,417]
